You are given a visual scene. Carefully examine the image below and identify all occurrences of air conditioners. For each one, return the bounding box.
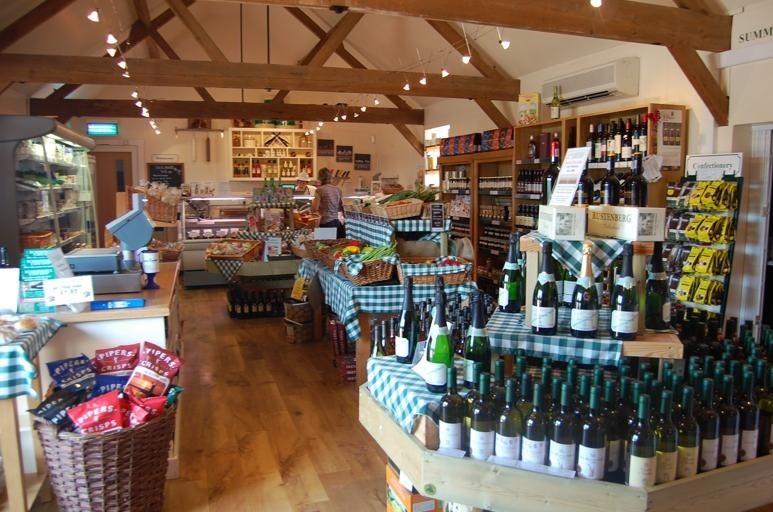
[540,54,641,107]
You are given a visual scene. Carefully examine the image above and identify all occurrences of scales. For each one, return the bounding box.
[63,206,156,294]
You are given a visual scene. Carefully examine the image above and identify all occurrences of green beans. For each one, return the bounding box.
[361,240,398,262]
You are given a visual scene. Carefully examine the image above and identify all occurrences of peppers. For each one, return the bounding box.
[333,239,373,258]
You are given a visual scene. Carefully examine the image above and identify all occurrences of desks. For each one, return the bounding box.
[0,317,68,512]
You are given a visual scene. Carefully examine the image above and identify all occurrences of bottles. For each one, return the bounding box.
[498,235,521,313]
[531,241,559,334]
[438,315,770,484]
[393,276,419,363]
[462,292,492,388]
[423,275,454,392]
[254,178,291,203]
[0,247,11,268]
[443,170,512,230]
[230,277,293,314]
[515,116,648,228]
[233,131,314,177]
[549,85,560,120]
[570,242,598,339]
[644,239,670,331]
[610,244,640,339]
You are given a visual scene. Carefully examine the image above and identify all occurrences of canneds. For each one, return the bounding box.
[0,246,9,267]
[442,169,512,194]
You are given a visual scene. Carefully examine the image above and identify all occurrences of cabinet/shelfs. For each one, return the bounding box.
[358,104,773,511]
[282,150,516,387]
[183,106,404,319]
[115,191,180,248]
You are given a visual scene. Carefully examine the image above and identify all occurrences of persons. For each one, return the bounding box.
[311,167,346,238]
[294,172,318,197]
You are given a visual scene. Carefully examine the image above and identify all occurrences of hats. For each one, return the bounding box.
[296,168,312,182]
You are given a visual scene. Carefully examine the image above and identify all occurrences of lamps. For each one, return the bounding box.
[399,26,511,91]
[86,4,162,139]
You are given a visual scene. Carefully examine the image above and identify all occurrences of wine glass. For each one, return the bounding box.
[140,250,161,289]
